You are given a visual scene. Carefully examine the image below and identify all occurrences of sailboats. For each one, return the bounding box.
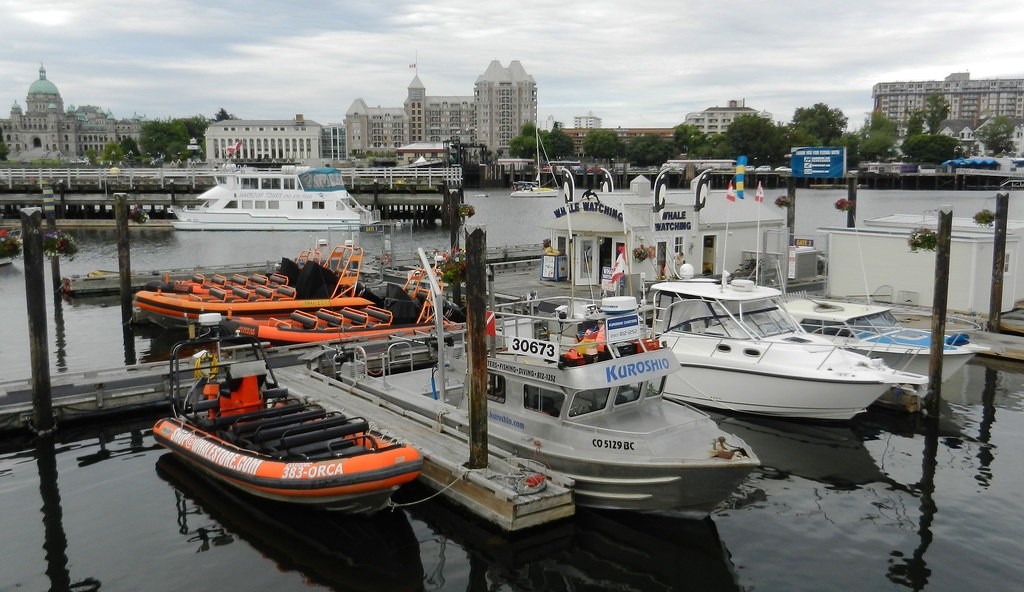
[509,123,560,197]
[755,220,991,412]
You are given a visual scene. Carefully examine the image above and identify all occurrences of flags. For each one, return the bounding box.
[754,182,765,203]
[226,141,242,154]
[408,64,416,68]
[611,245,626,284]
[726,181,736,202]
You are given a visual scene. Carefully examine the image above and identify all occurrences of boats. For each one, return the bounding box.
[485,204,931,422]
[341,204,764,522]
[189,256,463,352]
[168,140,381,232]
[62,231,382,330]
[152,311,422,515]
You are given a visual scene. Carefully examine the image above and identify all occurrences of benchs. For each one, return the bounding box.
[188,272,297,301]
[194,389,377,463]
[268,304,391,333]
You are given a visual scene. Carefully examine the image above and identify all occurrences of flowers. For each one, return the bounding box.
[635,246,648,261]
[776,194,789,209]
[908,230,938,251]
[46,231,79,257]
[459,203,473,216]
[543,239,551,244]
[837,199,854,212]
[441,247,467,275]
[977,211,997,225]
[132,205,150,219]
[0,229,22,258]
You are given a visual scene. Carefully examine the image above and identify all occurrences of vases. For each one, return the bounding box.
[838,205,852,212]
[53,240,69,253]
[463,210,475,216]
[912,239,936,250]
[1,242,17,258]
[638,254,646,261]
[777,202,788,208]
[979,215,992,227]
[545,243,549,248]
[137,217,146,224]
[443,270,467,286]
